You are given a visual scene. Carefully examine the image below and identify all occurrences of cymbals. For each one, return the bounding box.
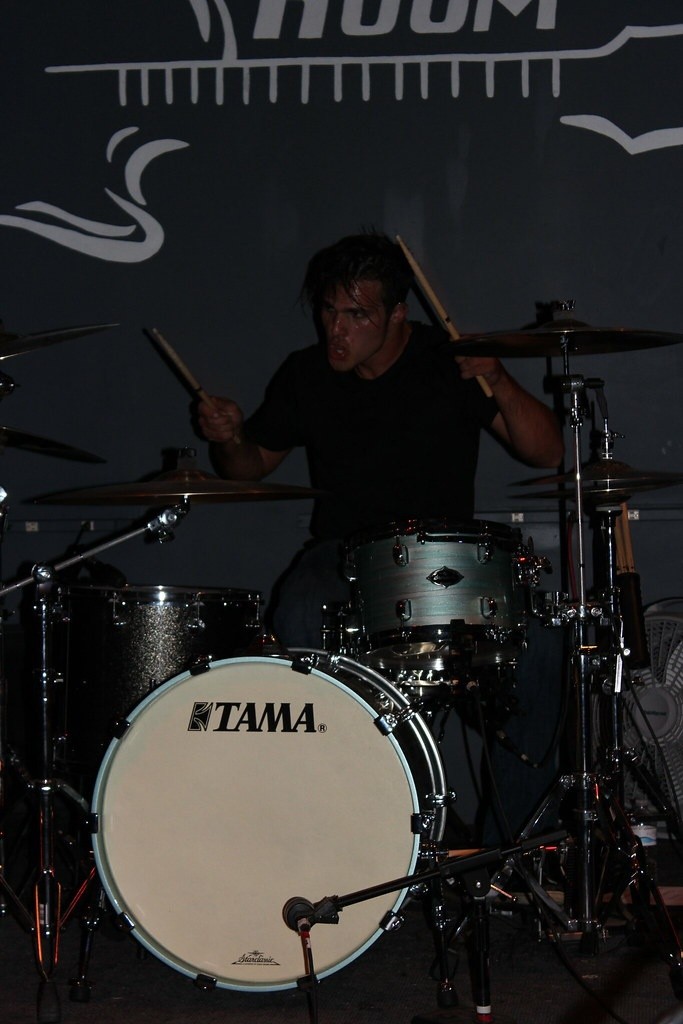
[434,318,683,358]
[503,458,683,506]
[24,468,335,506]
[0,322,121,360]
[0,424,108,464]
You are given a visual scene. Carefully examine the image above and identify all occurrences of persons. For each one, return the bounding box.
[195,234,564,901]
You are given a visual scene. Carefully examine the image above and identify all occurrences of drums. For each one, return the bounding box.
[90,647,450,994]
[338,517,528,673]
[53,581,274,781]
